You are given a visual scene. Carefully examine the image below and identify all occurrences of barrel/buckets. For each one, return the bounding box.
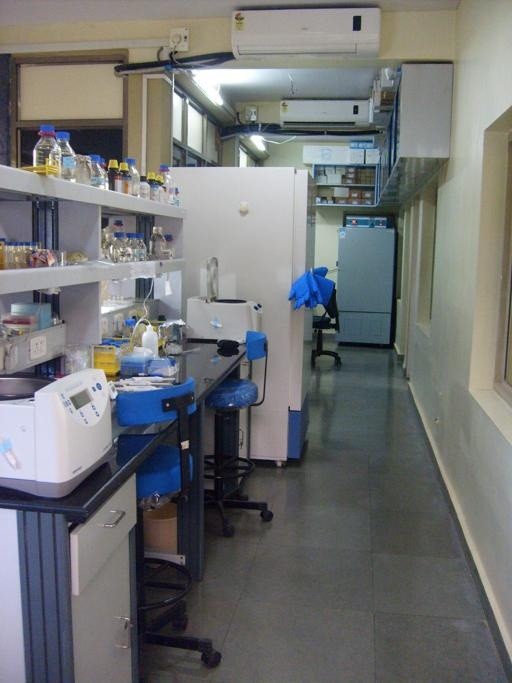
[141,506,178,554]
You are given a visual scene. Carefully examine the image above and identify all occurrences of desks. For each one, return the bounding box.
[0,334,254,683]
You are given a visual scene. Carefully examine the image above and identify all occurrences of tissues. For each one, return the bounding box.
[120,345,157,376]
[149,356,180,378]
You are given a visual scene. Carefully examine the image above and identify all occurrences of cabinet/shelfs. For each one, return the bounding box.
[377,61,454,209]
[0,164,188,373]
[312,164,377,208]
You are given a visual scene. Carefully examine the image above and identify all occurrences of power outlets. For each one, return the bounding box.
[30,336,49,361]
[169,26,190,52]
[244,103,259,123]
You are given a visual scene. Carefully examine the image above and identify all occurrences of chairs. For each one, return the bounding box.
[311,282,343,369]
[205,329,274,536]
[112,374,224,670]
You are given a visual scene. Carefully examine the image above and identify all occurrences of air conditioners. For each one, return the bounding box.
[231,4,382,60]
[281,97,373,133]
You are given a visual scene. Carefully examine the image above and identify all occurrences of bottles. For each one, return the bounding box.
[141,325,158,359]
[163,234,174,259]
[149,225,167,259]
[106,159,123,194]
[109,232,147,261]
[119,163,132,196]
[0,238,42,267]
[55,131,78,181]
[70,149,109,186]
[126,157,181,207]
[32,124,63,181]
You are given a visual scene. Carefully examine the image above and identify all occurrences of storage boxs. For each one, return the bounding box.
[315,165,376,205]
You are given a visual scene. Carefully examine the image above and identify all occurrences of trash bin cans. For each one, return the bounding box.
[141,503,180,570]
